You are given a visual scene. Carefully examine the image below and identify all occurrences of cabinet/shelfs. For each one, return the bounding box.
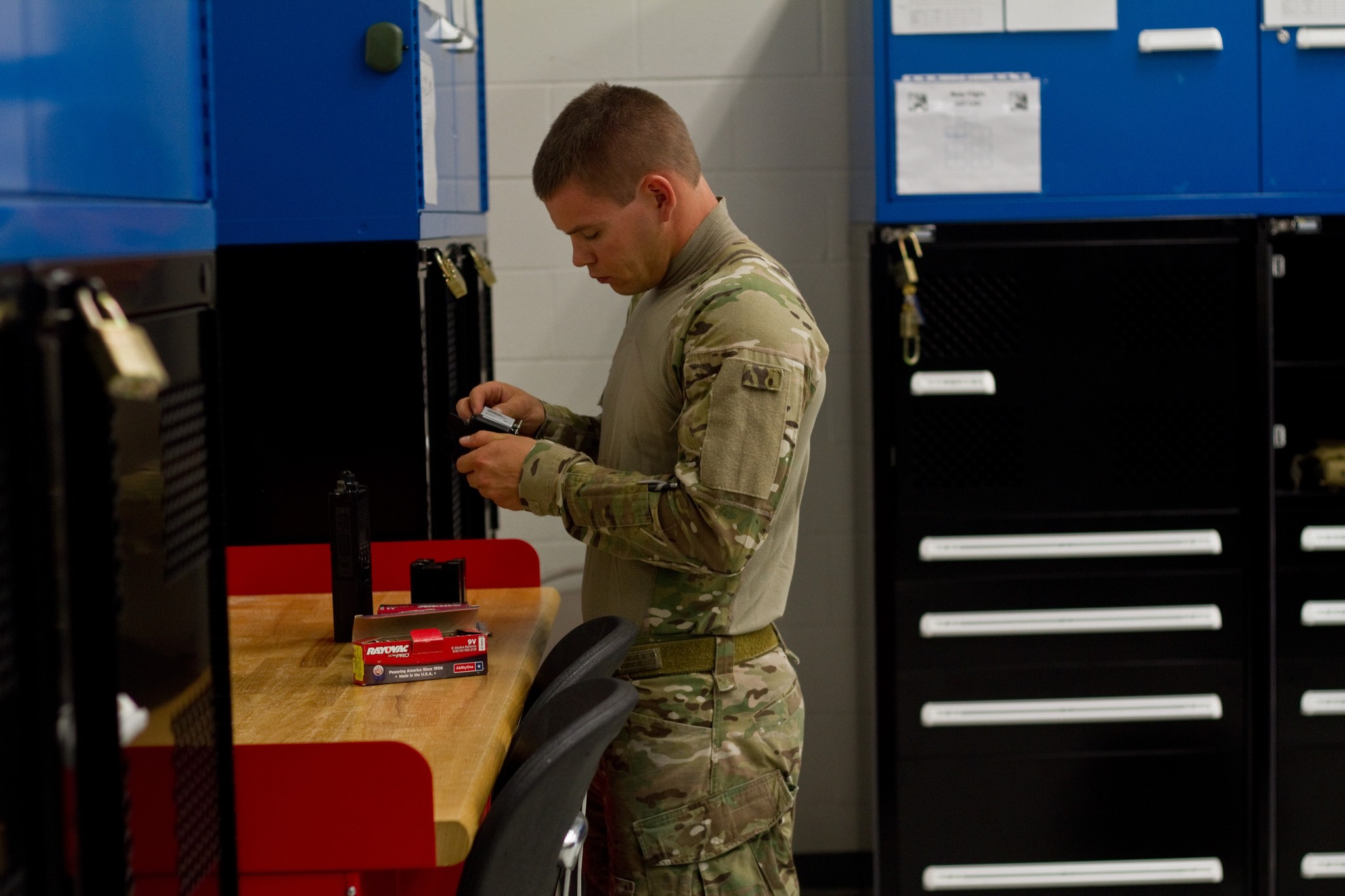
[0,0,491,265]
[865,1,1345,896]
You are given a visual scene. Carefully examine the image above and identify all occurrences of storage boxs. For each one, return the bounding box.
[352,605,488,686]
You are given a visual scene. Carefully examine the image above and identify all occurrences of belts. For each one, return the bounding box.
[613,624,780,679]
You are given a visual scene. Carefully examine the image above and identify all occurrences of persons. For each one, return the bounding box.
[457,83,831,896]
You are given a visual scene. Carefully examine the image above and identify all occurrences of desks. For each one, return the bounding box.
[123,538,561,896]
